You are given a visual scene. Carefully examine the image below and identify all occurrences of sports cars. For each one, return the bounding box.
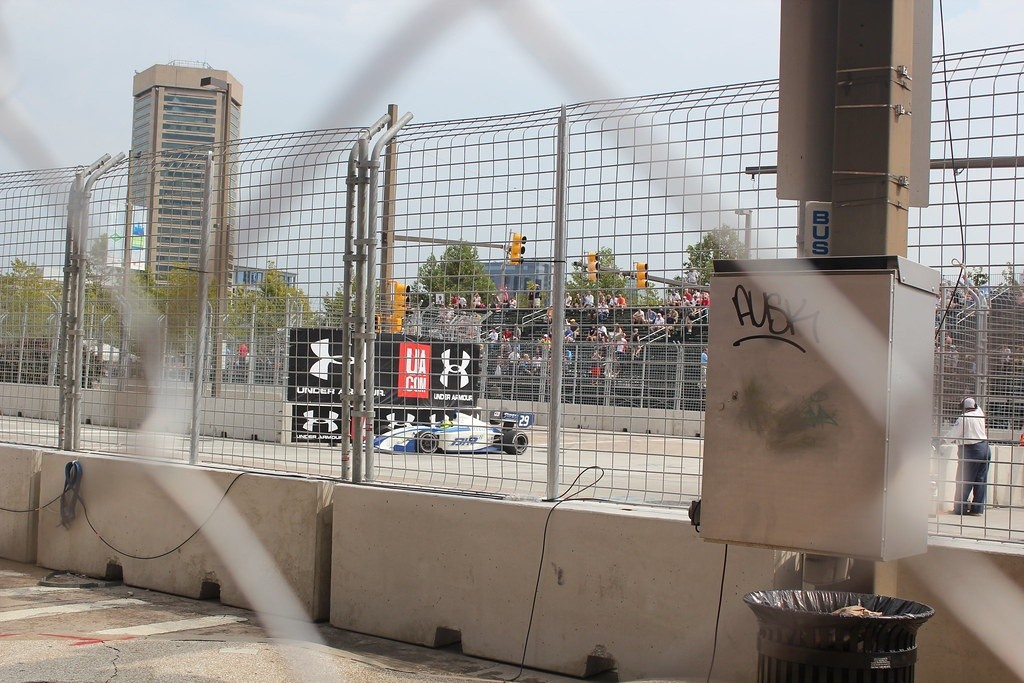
[373,412,529,455]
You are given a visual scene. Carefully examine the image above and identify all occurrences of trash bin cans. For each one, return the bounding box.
[743,590,935,683]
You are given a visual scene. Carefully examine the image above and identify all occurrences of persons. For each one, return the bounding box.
[941,397,992,516]
[217,270,1024,387]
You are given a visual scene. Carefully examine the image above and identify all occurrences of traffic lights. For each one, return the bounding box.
[509,232,527,266]
[390,280,410,332]
[586,251,600,282]
[635,262,650,290]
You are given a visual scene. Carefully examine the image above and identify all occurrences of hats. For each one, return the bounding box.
[543,334,548,337]
[569,319,575,322]
[958,397,977,409]
[683,296,687,299]
[657,313,662,316]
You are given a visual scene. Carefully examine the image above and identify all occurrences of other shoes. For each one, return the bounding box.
[947,510,983,516]
